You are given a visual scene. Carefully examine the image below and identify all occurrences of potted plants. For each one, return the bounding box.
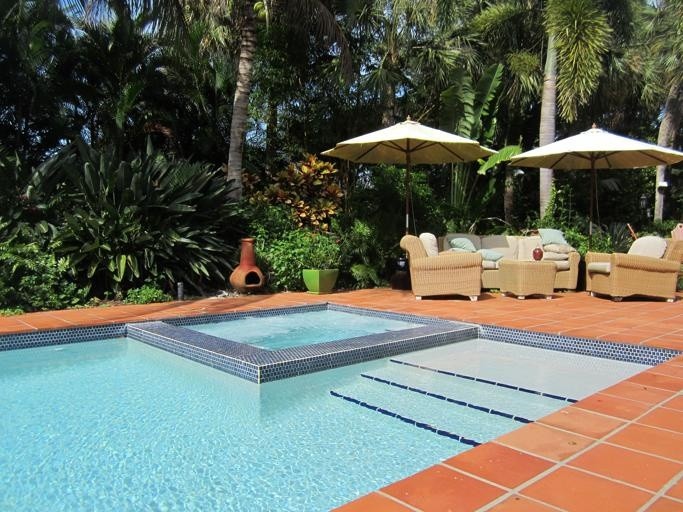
[293,235,344,294]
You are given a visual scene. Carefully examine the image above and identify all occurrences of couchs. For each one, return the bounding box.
[437,236,581,292]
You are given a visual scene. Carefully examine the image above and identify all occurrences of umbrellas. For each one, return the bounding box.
[320,117,499,236]
[508,121,683,253]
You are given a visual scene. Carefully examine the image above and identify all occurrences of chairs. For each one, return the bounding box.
[400,234,483,302]
[585,240,683,302]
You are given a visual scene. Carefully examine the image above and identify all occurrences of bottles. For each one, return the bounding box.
[533,248,543,261]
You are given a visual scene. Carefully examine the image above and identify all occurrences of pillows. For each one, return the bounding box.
[443,228,576,260]
[628,236,667,259]
[420,232,438,257]
